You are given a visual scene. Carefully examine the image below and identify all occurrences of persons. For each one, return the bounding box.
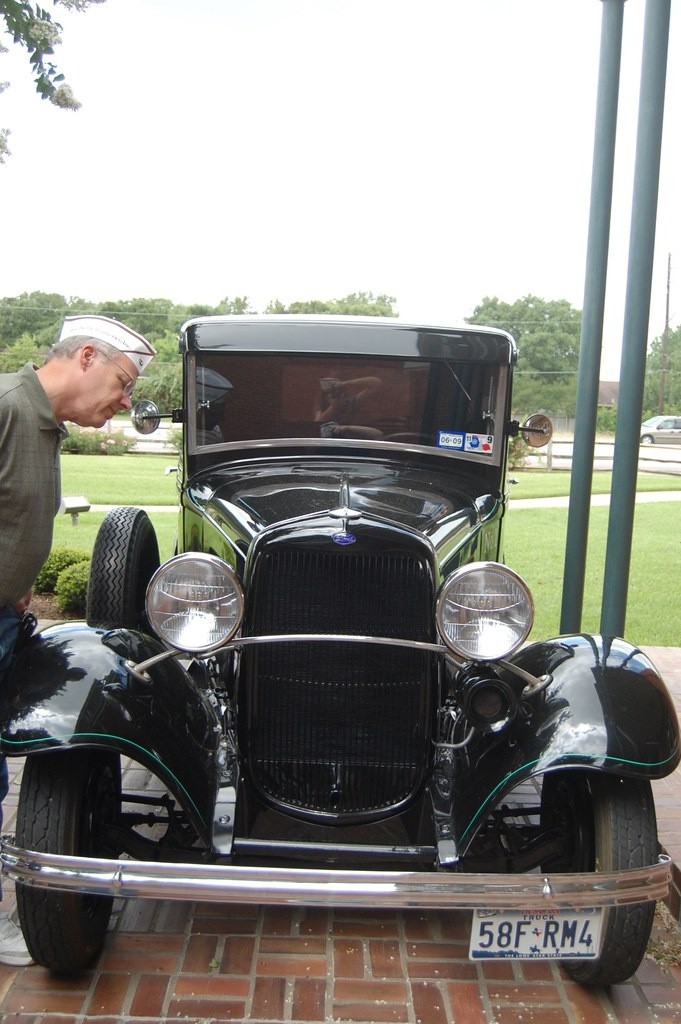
[0,315,157,965]
[314,370,383,440]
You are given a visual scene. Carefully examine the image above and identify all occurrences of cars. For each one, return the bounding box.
[0,312,680,987]
[639,415,681,447]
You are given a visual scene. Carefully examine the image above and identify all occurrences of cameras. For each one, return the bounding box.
[331,384,341,398]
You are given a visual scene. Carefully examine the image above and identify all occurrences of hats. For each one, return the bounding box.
[58,314,157,377]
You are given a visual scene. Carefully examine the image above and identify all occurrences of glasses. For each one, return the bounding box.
[94,349,135,396]
